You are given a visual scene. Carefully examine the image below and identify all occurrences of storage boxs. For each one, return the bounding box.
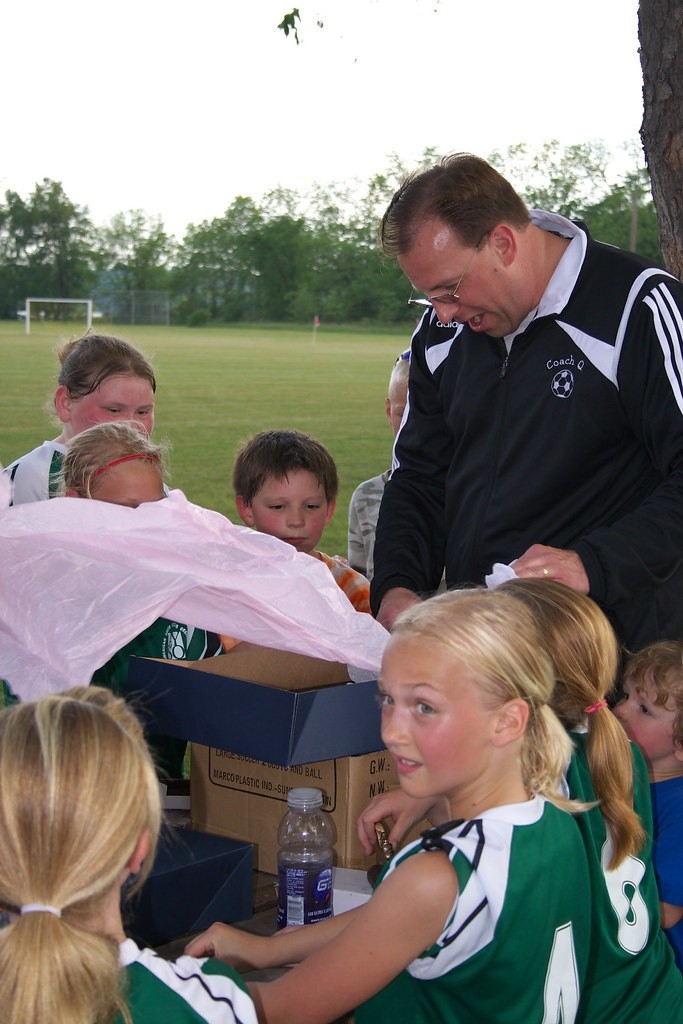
[120,652,437,878]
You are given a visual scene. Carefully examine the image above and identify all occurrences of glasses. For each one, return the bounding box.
[395,350,410,366]
[408,229,490,308]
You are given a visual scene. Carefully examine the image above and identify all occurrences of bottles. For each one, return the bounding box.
[276,788,334,934]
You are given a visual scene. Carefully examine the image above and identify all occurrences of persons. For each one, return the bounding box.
[369,150,683,709]
[0,334,683,1024]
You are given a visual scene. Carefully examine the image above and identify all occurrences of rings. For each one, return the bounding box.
[541,567,548,578]
